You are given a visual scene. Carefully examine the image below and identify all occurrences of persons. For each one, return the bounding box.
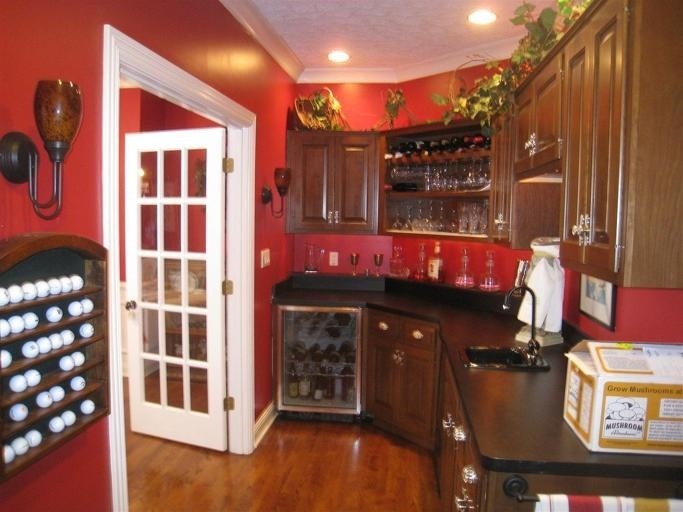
[370,87,407,130]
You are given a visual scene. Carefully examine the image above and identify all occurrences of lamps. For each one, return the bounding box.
[0,79,82,220]
[260,167,292,218]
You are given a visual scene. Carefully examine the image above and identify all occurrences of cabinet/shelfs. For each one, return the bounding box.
[0,234,109,484]
[377,36,563,244]
[558,0,683,289]
[286,131,378,235]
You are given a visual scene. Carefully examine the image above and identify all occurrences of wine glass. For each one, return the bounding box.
[350,254,359,278]
[374,253,385,281]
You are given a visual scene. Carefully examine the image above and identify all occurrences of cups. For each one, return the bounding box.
[304,242,325,273]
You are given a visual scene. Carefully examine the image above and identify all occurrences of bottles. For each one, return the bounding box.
[428,241,445,282]
[389,245,407,277]
[478,250,500,292]
[286,340,355,402]
[389,159,490,192]
[454,248,475,289]
[387,132,490,156]
[415,239,426,280]
[391,199,489,233]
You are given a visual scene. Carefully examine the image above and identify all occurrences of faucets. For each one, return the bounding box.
[501,285,540,355]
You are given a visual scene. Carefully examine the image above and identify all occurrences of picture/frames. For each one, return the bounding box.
[577,273,616,332]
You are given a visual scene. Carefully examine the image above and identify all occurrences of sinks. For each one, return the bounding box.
[457,343,552,371]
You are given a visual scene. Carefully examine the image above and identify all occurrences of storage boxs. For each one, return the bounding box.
[559,338,683,454]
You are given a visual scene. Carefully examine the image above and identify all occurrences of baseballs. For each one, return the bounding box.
[0,275,94,464]
